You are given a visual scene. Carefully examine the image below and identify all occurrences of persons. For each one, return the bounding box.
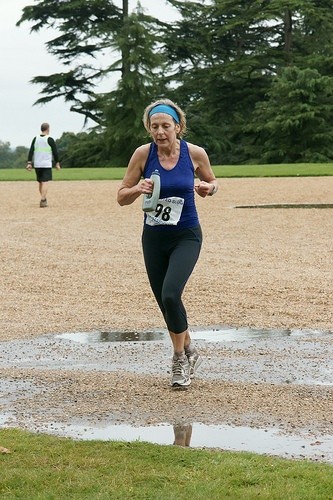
[26,123,61,208]
[116,98,218,390]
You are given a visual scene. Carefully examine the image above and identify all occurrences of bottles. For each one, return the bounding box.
[142,168,160,212]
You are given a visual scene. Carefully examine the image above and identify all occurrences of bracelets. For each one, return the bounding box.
[207,184,215,196]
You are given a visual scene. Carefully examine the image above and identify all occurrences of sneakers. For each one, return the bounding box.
[171,348,203,387]
[39,198,47,207]
